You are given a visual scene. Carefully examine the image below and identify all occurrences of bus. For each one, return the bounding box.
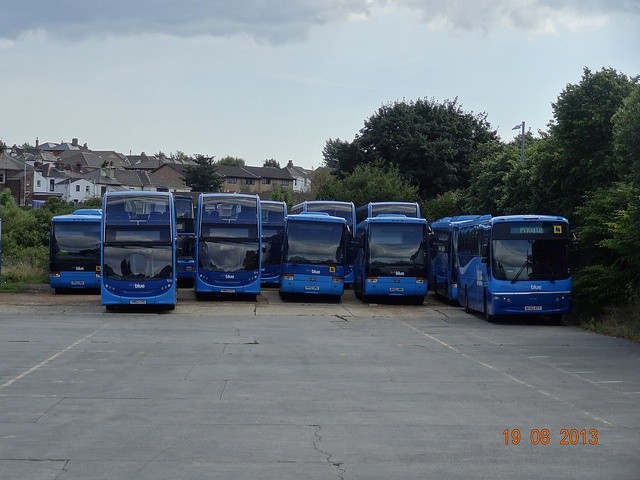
[358,214,433,296]
[50,209,102,287]
[195,193,262,294]
[425,214,492,303]
[171,193,196,279]
[291,200,357,283]
[451,215,576,322]
[280,212,347,296]
[260,200,287,283]
[358,202,421,219]
[100,191,176,305]
